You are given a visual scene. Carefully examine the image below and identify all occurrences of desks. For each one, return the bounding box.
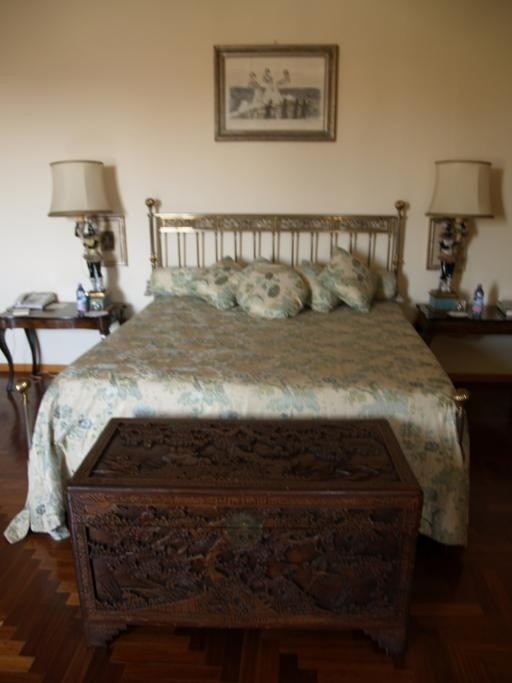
[1,307,124,392]
[413,301,512,362]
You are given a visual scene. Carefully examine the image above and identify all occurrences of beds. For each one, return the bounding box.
[6,197,469,545]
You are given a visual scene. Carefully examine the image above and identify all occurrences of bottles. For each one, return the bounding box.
[472,284,484,320]
[76,283,89,311]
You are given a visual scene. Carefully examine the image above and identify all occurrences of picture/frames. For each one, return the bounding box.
[214,43,339,142]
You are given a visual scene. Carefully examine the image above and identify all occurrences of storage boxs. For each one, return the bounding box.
[67,416,423,654]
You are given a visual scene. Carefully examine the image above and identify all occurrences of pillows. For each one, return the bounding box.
[296,260,341,313]
[238,264,307,317]
[370,264,395,300]
[315,247,376,314]
[149,263,206,296]
[184,254,247,310]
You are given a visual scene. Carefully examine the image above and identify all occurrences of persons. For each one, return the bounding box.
[249,67,293,108]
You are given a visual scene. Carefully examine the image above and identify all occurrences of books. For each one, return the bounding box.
[496,298,512,316]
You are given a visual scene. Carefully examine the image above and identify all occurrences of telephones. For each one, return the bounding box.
[12,291,58,311]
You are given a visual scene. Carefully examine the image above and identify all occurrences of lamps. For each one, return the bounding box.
[426,158,494,311]
[48,159,113,309]
